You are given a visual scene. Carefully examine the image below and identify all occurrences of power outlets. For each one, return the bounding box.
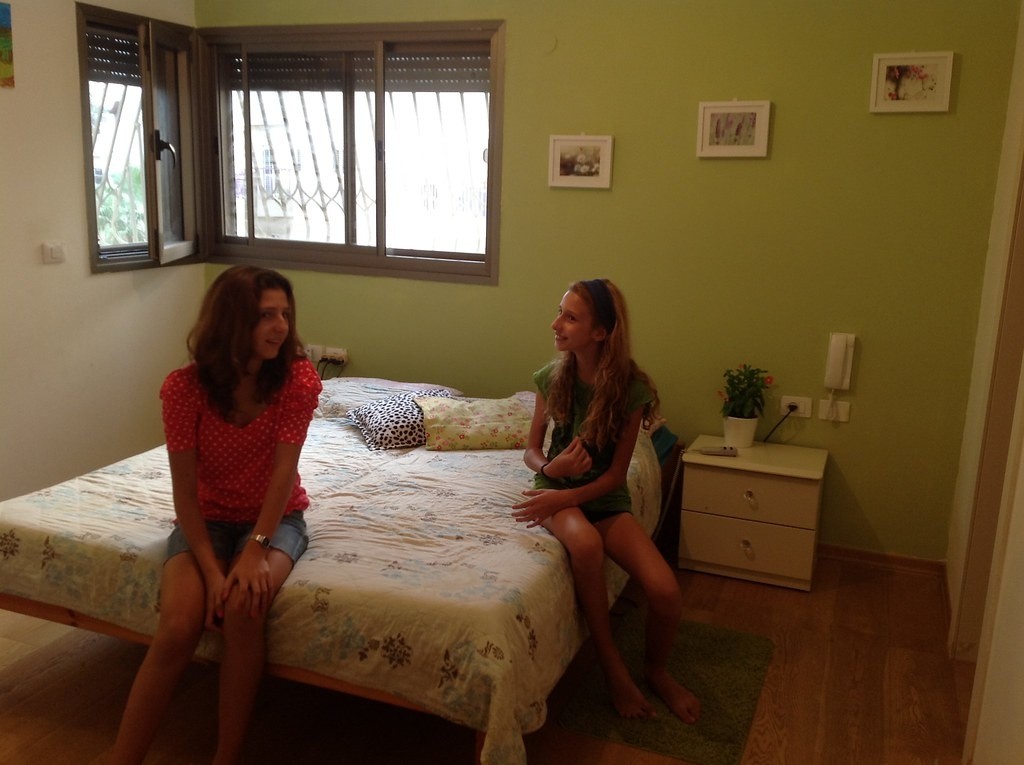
[308,344,322,362]
[780,395,812,417]
[325,347,348,365]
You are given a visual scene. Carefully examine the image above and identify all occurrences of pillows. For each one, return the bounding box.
[413,392,555,449]
[348,389,454,450]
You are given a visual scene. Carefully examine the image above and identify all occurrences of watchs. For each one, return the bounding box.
[245,534,272,550]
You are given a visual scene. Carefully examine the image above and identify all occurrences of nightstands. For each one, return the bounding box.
[675,434,829,590]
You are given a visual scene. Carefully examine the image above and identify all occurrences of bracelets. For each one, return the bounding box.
[541,464,547,475]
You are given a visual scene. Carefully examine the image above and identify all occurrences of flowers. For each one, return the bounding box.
[715,363,771,418]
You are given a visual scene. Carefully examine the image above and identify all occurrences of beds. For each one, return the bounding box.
[0,376,673,765]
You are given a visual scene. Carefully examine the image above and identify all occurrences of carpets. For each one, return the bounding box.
[555,605,774,765]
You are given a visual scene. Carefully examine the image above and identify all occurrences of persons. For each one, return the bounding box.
[108,265,323,763]
[510,277,705,725]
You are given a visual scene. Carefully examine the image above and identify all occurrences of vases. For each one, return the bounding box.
[723,415,760,449]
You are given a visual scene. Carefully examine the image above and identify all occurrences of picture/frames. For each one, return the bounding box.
[868,52,955,113]
[546,134,614,189]
[697,98,770,157]
